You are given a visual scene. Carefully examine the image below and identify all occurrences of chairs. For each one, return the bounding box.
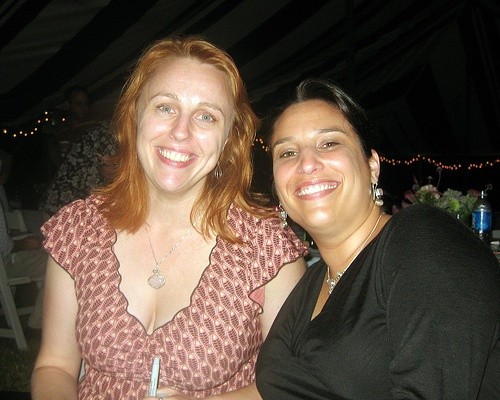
[0,234,47,353]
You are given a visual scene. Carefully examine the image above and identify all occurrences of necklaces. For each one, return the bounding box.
[327,211,386,295]
[145,212,207,291]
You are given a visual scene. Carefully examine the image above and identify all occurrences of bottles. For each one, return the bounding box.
[471,189,492,247]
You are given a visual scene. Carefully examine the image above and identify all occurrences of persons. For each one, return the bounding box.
[29,38,312,400]
[255,79,500,400]
[27,87,90,212]
[25,118,121,335]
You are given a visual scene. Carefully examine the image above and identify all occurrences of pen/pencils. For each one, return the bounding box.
[149,357,160,397]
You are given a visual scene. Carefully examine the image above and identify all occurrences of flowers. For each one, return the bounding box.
[401,169,477,221]
